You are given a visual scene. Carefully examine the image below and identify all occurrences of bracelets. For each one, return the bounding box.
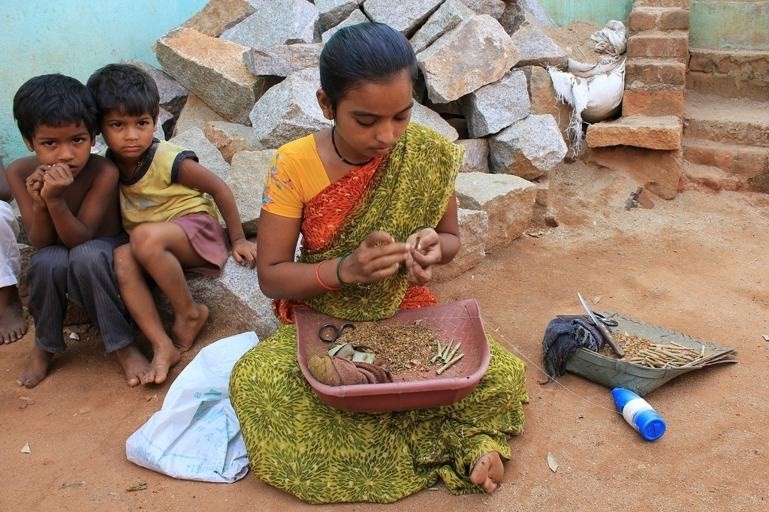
[335,252,353,285]
[315,258,342,291]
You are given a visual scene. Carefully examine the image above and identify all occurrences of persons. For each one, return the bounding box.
[226,22,533,504]
[84,63,259,386]
[0,158,29,347]
[6,72,151,389]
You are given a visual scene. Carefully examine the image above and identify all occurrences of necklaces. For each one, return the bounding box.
[331,125,375,167]
